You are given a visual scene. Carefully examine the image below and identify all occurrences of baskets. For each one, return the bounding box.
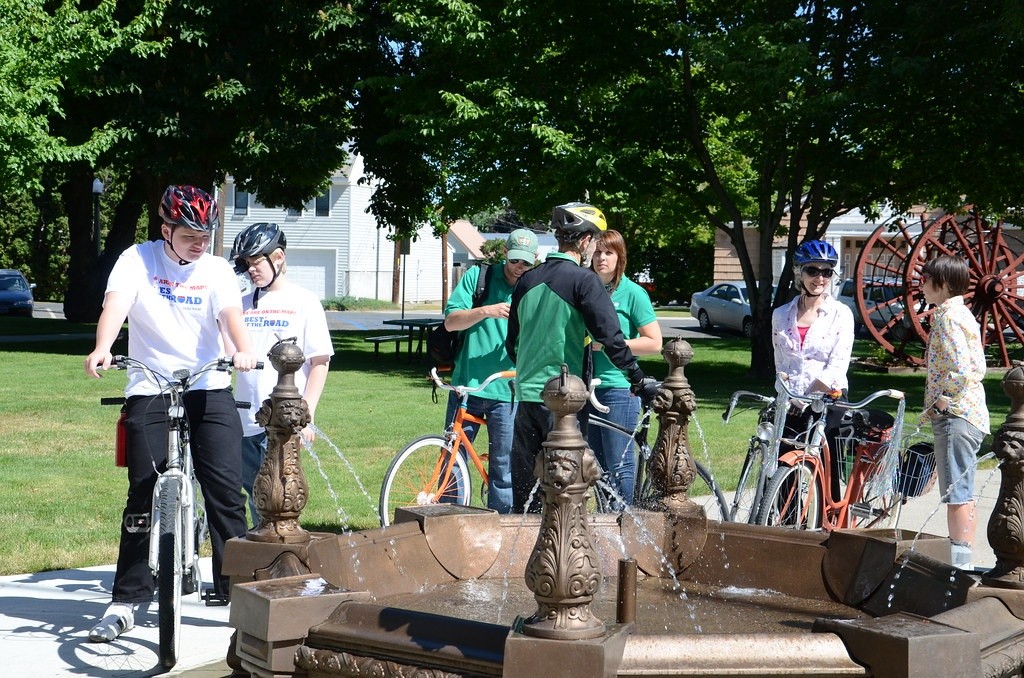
[835,417,939,498]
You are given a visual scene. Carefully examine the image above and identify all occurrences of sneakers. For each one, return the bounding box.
[87,605,135,643]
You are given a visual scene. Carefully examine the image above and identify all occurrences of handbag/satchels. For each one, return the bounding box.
[426,261,492,365]
[895,442,935,498]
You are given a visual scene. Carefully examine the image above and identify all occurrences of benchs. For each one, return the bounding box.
[364,334,417,363]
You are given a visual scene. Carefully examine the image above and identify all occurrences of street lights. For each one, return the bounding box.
[93,177,105,258]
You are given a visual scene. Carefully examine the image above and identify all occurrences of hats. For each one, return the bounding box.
[504,228,539,265]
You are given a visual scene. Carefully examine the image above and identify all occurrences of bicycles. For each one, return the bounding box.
[721,389,808,527]
[756,371,938,532]
[377,365,516,527]
[98,356,266,670]
[585,377,731,525]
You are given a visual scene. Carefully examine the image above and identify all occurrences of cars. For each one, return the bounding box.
[0,267,38,316]
[689,280,779,341]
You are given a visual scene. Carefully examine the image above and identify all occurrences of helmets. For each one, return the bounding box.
[227,222,288,262]
[156,183,220,234]
[549,200,608,244]
[791,240,839,275]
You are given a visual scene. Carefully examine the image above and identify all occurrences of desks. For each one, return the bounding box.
[383,319,445,364]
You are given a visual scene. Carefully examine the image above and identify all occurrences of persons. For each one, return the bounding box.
[772,241,854,524]
[438,229,538,514]
[590,230,662,503]
[920,256,991,548]
[84,186,257,642]
[505,202,657,512]
[219,223,334,525]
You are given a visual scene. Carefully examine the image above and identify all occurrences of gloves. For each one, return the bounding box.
[630,370,667,402]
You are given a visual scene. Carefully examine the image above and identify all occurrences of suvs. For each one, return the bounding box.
[837,274,930,342]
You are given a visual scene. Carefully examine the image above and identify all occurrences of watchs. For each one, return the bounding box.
[932,404,943,415]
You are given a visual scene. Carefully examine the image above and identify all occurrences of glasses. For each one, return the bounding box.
[919,274,938,286]
[799,265,834,279]
[507,258,533,267]
